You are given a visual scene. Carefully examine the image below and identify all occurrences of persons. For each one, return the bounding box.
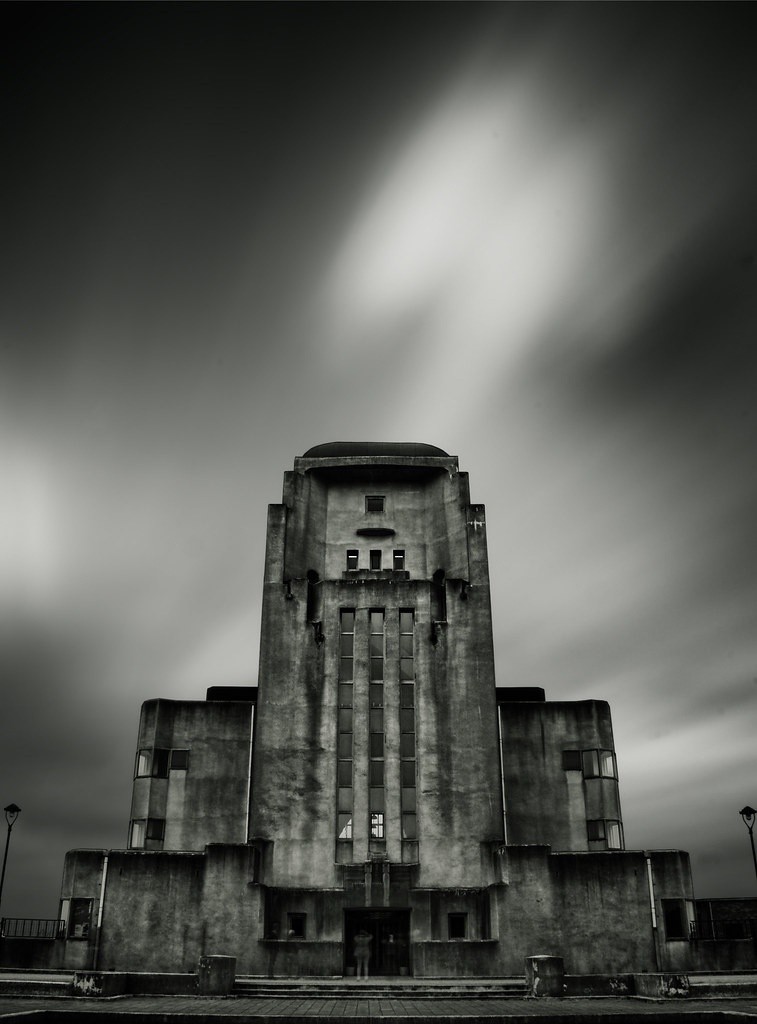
[354,930,372,981]
[265,924,280,980]
[284,929,305,979]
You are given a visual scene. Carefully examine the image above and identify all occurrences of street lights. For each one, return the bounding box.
[738,806,757,871]
[0,803,21,904]
